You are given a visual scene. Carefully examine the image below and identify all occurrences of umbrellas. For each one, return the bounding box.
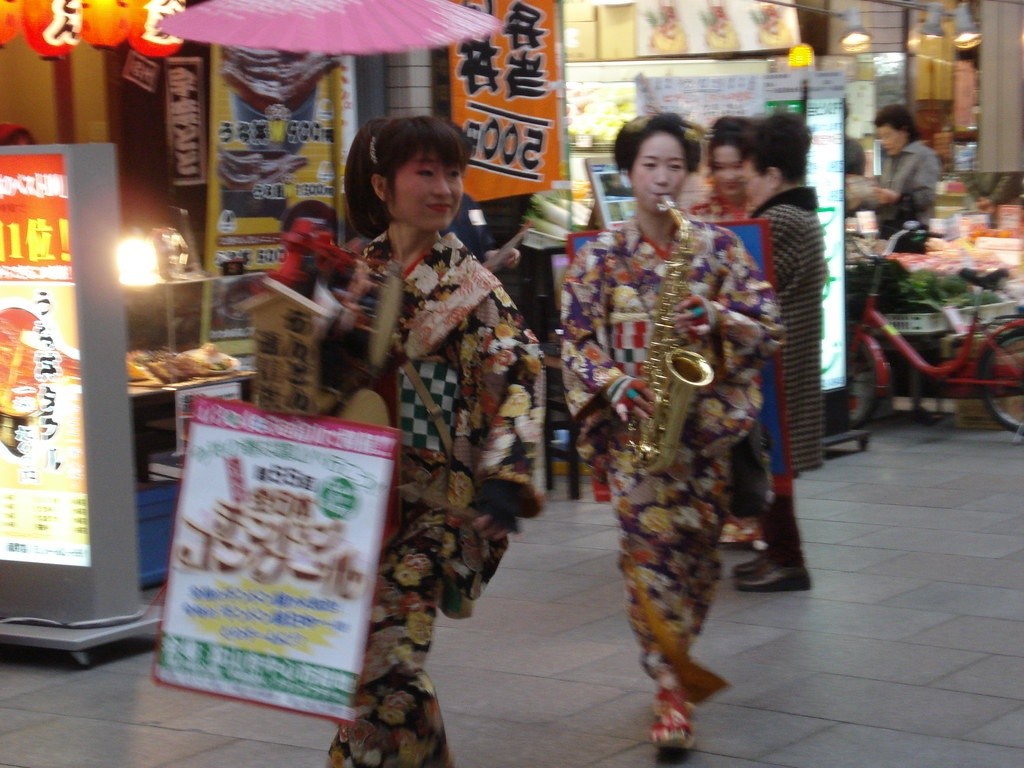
[154,1,504,243]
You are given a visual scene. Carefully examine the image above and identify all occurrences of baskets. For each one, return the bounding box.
[877,301,1013,337]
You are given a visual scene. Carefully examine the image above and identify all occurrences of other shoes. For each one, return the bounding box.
[652,685,696,749]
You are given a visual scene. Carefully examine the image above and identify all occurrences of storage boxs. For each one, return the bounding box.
[884,301,1023,334]
[136,481,180,585]
[939,335,1024,429]
[126,271,261,588]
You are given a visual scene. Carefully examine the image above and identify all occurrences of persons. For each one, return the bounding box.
[691,114,760,552]
[844,99,865,173]
[554,107,788,756]
[855,102,941,411]
[310,115,547,768]
[439,190,521,270]
[731,111,830,593]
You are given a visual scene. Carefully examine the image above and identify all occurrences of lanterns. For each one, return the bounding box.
[0,0,191,61]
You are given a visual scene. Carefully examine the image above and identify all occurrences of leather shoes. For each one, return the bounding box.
[740,563,808,592]
[735,555,769,575]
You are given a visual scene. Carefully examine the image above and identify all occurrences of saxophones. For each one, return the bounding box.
[629,194,714,480]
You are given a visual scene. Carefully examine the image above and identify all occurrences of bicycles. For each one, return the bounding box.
[844,223,1024,438]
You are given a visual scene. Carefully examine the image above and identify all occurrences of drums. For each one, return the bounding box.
[315,256,405,371]
[319,384,390,430]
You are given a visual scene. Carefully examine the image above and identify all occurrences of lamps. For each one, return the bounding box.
[915,3,946,38]
[953,3,983,42]
[839,9,873,46]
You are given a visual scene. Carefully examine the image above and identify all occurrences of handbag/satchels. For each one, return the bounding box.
[727,416,784,516]
[449,462,508,620]
